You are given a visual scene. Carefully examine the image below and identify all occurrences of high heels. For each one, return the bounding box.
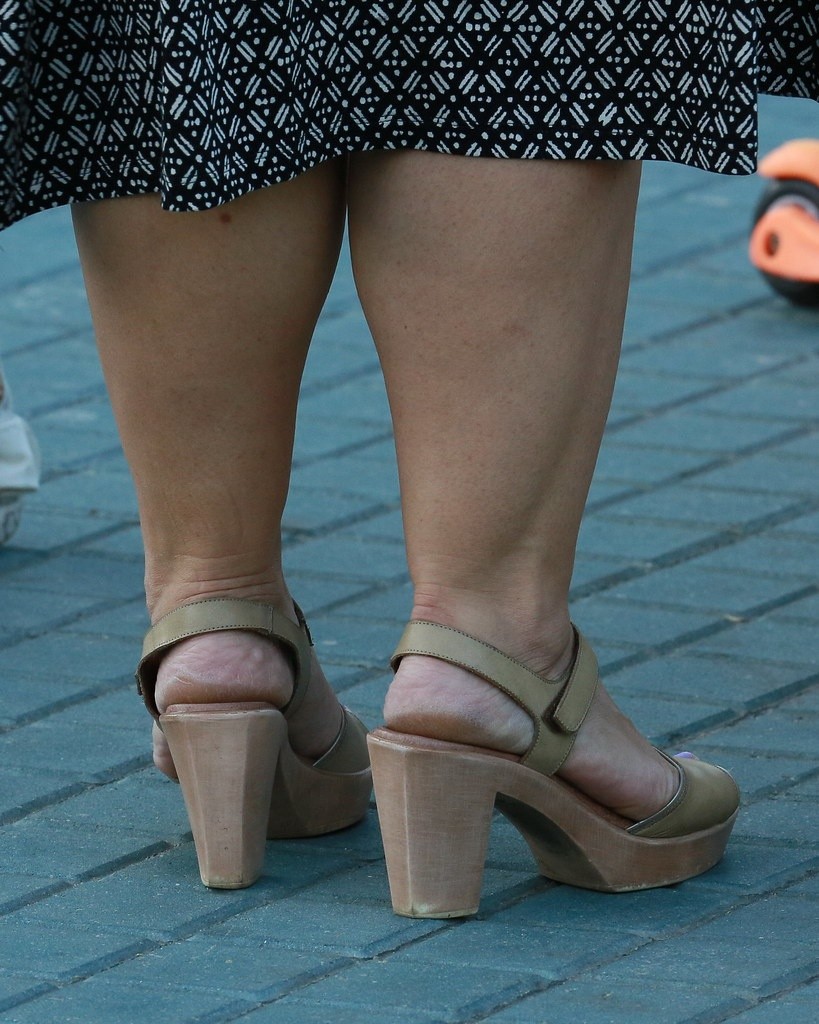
[134,601,372,891]
[365,617,741,920]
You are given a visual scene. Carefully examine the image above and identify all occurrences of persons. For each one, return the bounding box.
[0,1,819,921]
